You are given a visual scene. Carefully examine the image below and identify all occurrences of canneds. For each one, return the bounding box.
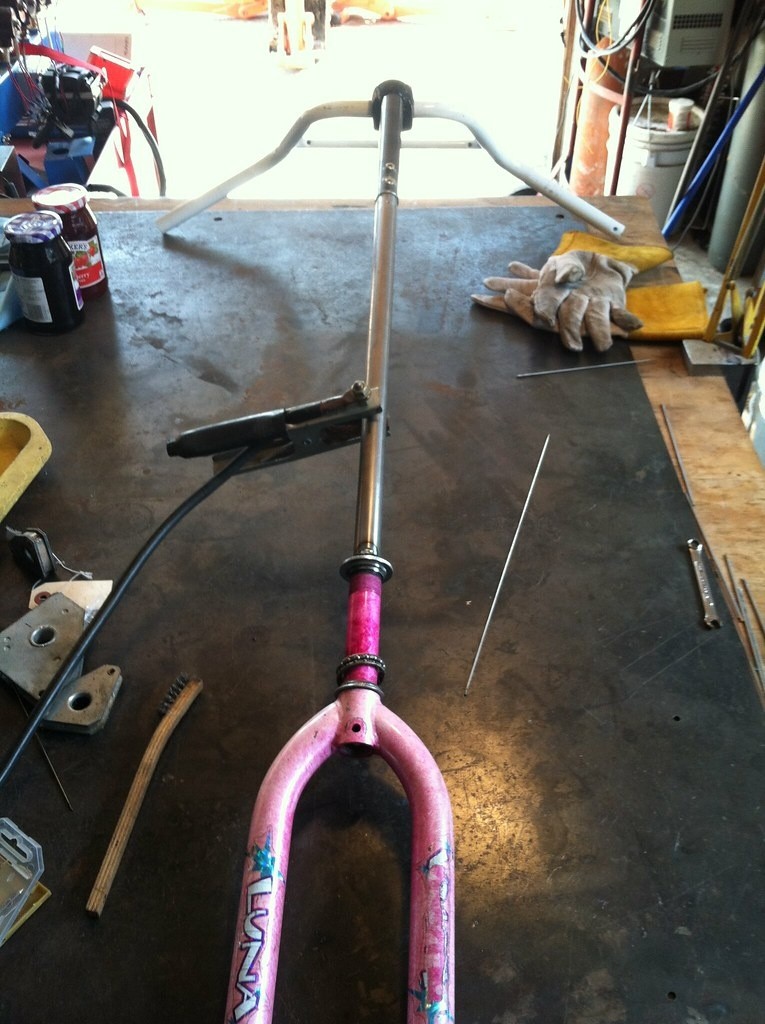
[3,182,108,335]
[667,98,693,132]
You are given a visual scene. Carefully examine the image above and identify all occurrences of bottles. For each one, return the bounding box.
[665,97,695,133]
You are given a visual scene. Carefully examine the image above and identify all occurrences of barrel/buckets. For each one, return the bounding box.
[603,96,707,239]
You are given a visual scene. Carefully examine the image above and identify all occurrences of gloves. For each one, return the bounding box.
[534,231,674,353]
[471,262,710,341]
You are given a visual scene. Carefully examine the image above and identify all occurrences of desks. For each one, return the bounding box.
[1,198,764,1024]
